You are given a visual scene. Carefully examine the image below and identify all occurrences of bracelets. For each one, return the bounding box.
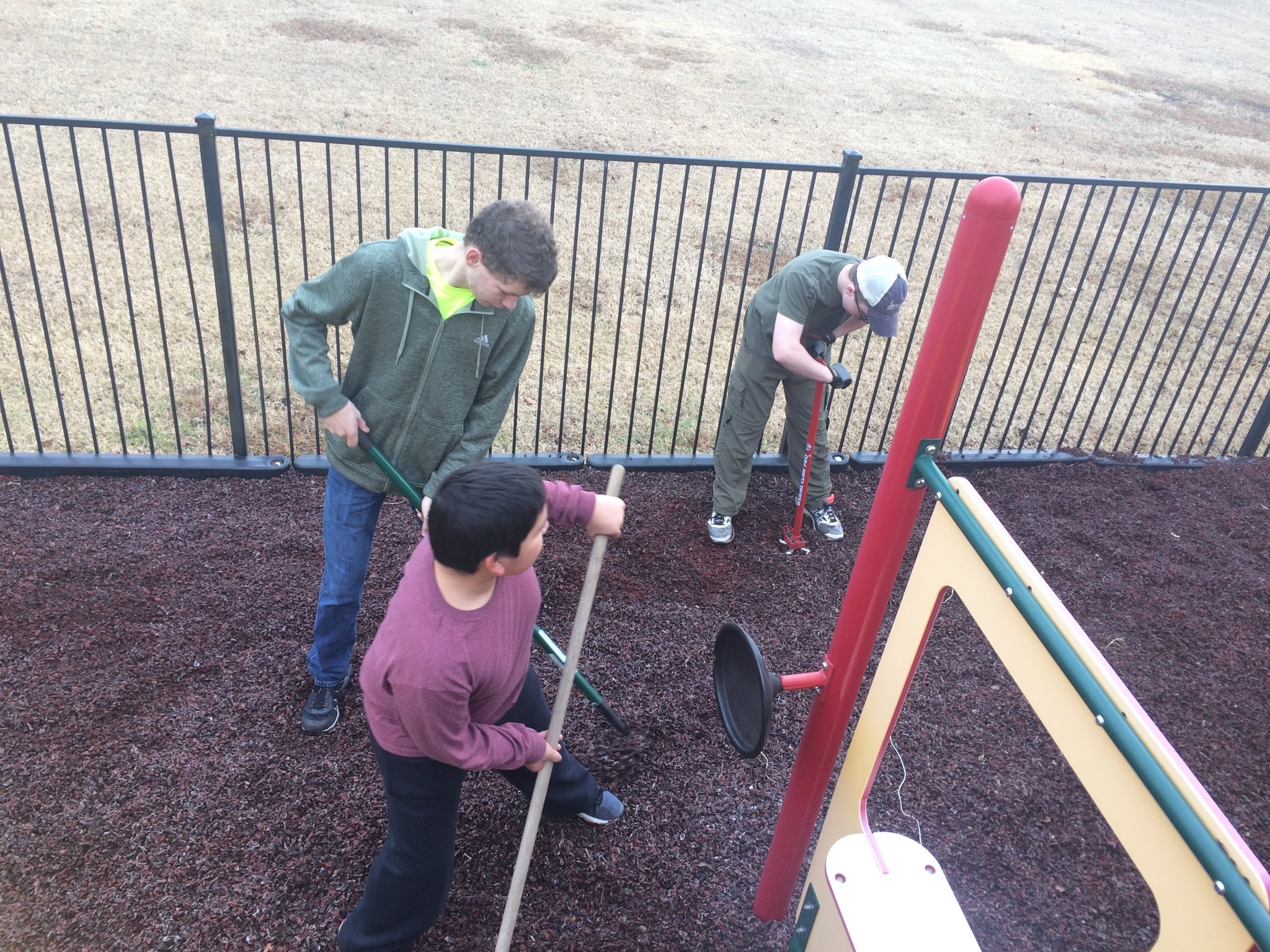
[827,332,836,345]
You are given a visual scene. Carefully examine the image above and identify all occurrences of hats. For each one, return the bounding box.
[857,254,909,337]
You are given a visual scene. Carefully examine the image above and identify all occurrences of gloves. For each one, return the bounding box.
[828,362,852,389]
[807,333,837,359]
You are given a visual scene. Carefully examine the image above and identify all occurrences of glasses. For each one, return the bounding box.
[854,281,868,323]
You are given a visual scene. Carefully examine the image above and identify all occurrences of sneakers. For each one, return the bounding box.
[576,784,623,824]
[706,504,734,544]
[301,664,352,735]
[803,505,844,541]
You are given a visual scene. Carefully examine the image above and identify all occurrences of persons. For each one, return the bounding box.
[279,200,559,733]
[335,463,626,952]
[707,249,909,545]
